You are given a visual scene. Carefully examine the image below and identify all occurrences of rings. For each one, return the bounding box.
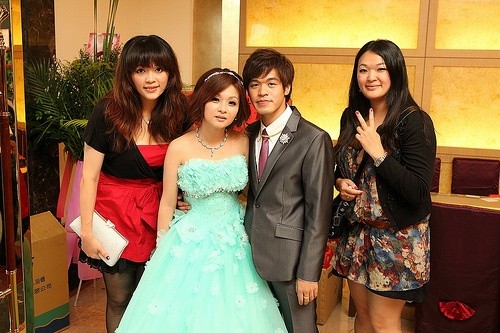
[303,296,309,299]
[347,194,350,199]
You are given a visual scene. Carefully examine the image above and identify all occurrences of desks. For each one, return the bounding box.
[415,192,500,333]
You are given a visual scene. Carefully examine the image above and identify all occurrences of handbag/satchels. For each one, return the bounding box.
[69,208,129,267]
[328,194,348,238]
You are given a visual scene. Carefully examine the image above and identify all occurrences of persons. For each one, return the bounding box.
[331,39,437,333]
[177,48,336,333]
[156,67,249,333]
[80,35,194,333]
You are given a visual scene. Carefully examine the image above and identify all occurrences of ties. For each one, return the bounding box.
[258,128,270,179]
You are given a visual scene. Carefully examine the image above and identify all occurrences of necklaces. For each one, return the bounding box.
[195,126,228,158]
[142,115,151,125]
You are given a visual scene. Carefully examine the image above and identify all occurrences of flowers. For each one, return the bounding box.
[23,0,122,161]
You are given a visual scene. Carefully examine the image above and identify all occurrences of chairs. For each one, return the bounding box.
[451,158,500,196]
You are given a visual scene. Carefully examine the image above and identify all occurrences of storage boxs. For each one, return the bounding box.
[29,211,70,333]
[315,268,415,325]
